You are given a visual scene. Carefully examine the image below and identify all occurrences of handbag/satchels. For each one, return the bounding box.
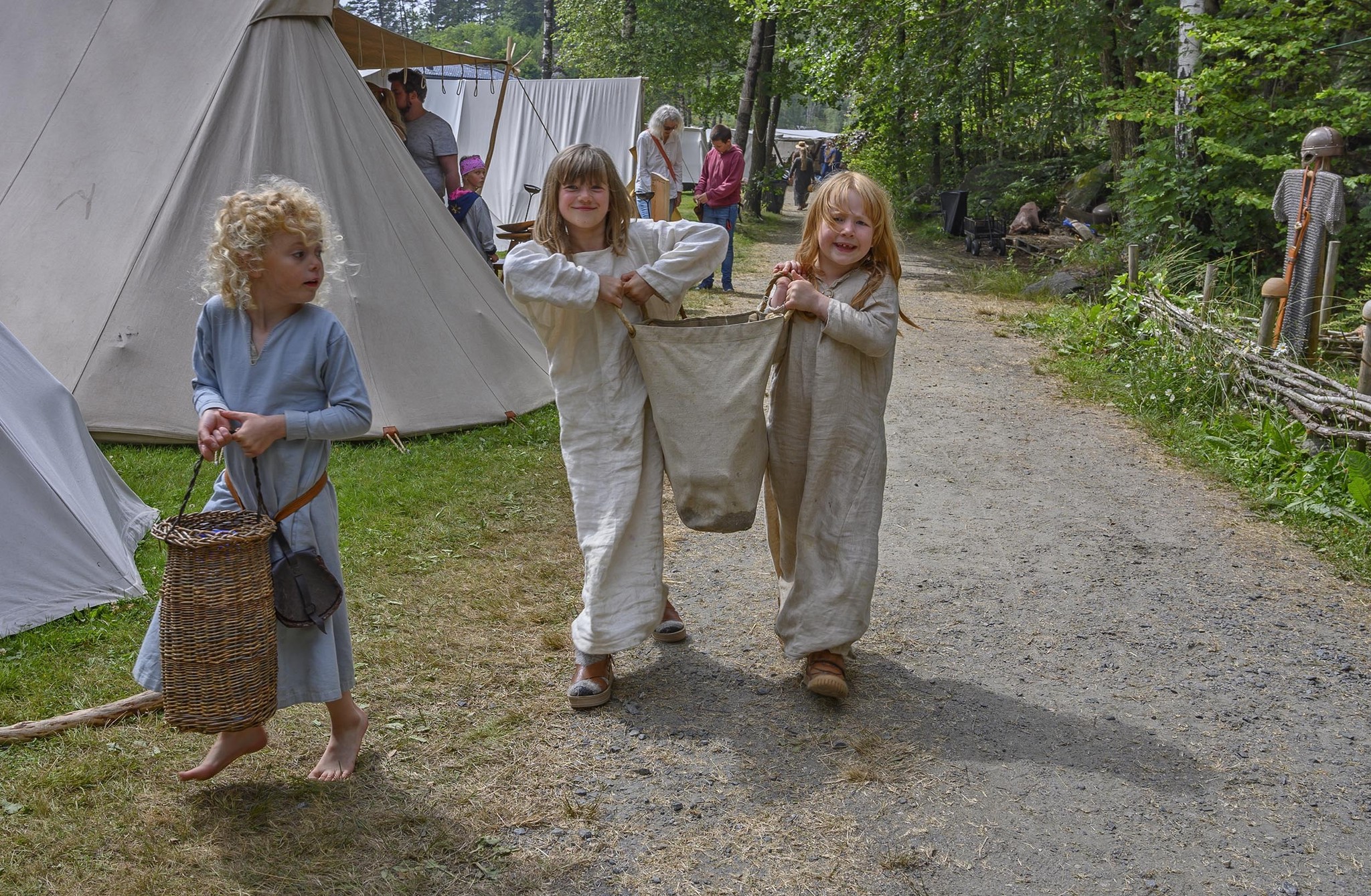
[272,548,343,635]
[671,207,682,221]
[614,270,794,534]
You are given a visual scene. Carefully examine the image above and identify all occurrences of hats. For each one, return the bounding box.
[796,141,809,149]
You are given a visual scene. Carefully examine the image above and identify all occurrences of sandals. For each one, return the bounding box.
[567,652,615,707]
[651,601,686,642]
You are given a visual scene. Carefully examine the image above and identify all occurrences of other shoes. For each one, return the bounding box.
[797,208,802,211]
[804,650,848,699]
[803,204,808,208]
[694,283,710,290]
[722,283,735,293]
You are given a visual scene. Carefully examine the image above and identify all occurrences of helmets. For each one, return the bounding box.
[1300,122,1346,164]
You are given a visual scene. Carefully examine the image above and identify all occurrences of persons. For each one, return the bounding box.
[502,144,730,709]
[133,169,372,781]
[757,171,923,697]
[366,82,406,142]
[448,154,499,271]
[387,68,461,206]
[693,124,745,293]
[635,104,684,222]
[789,137,842,210]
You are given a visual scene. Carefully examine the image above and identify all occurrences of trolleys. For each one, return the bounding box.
[963,196,1007,257]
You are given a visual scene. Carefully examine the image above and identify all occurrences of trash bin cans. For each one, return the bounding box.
[937,190,968,235]
[766,179,787,214]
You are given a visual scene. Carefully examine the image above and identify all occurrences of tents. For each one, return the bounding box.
[0,0,555,443]
[0,330,160,641]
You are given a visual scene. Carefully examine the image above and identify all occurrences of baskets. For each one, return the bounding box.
[150,433,279,734]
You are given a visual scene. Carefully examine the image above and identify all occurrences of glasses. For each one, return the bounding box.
[663,125,678,130]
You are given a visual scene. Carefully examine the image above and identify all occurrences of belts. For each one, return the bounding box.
[223,471,328,522]
[737,203,744,210]
